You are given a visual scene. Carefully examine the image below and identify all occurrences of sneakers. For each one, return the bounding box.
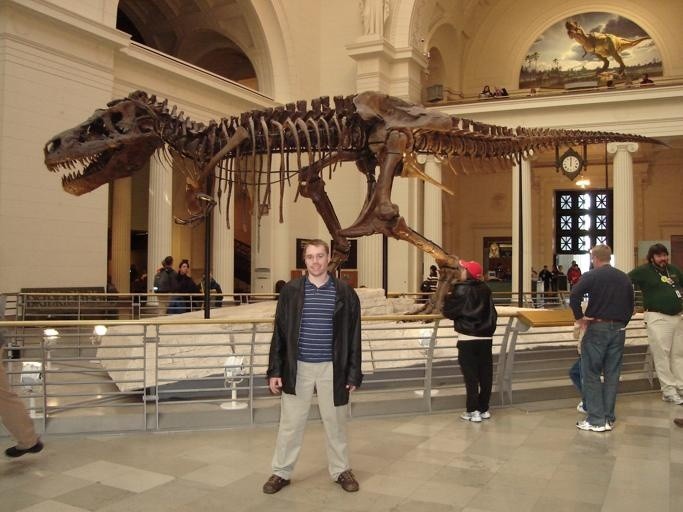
[577,401,589,414]
[576,420,605,432]
[606,418,614,430]
[337,470,359,491]
[263,475,289,493]
[662,393,683,404]
[461,410,482,422]
[480,410,491,419]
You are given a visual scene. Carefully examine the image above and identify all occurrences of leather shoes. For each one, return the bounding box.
[6,441,43,457]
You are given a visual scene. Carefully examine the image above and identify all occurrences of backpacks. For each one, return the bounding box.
[154,268,174,293]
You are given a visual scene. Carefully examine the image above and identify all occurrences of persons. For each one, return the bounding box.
[0,292,44,457]
[135,257,222,314]
[537,260,583,302]
[569,357,589,414]
[425,264,438,301]
[501,87,510,97]
[442,259,496,423]
[639,73,654,86]
[571,245,634,431]
[264,239,362,494]
[478,85,492,96]
[626,245,682,422]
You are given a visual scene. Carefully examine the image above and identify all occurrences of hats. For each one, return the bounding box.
[460,260,483,281]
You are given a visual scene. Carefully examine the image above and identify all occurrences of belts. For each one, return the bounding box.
[593,318,621,323]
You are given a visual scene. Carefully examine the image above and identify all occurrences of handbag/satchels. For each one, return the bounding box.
[168,296,187,313]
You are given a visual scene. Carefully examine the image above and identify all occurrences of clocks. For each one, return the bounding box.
[559,148,583,181]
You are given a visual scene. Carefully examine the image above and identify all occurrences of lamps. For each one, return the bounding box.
[414,330,438,397]
[221,357,248,409]
[20,360,47,418]
[41,328,60,371]
[90,325,106,346]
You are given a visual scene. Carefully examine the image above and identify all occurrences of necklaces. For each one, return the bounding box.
[655,267,674,286]
